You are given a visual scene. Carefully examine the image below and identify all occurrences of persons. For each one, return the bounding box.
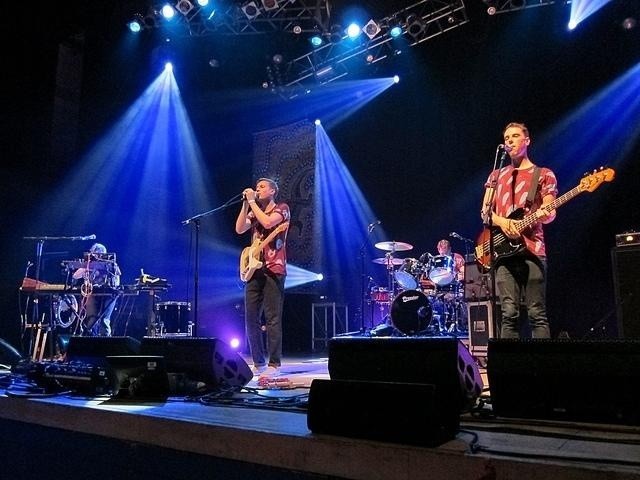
[480,121,558,339]
[235,177,291,380]
[436,240,465,282]
[73,243,121,337]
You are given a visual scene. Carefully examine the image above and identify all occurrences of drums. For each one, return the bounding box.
[396,257,422,289]
[155,301,192,337]
[389,290,434,336]
[428,254,454,286]
[371,288,393,303]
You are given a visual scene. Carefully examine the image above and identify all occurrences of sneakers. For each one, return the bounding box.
[246,364,269,376]
[259,366,282,378]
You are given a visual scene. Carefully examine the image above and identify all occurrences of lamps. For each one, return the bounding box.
[318,272,335,283]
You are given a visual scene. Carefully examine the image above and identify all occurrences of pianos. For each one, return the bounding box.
[19,279,173,295]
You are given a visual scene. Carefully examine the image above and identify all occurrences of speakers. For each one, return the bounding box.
[65,332,141,366]
[328,336,483,413]
[142,335,254,391]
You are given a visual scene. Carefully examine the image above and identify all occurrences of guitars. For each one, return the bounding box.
[239,223,288,283]
[475,166,615,268]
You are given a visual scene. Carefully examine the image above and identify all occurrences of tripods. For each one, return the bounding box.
[337,249,373,339]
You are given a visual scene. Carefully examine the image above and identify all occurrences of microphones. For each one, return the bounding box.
[450,231,463,241]
[371,219,381,228]
[81,232,96,243]
[504,143,514,153]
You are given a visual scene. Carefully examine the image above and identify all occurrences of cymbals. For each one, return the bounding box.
[374,241,413,251]
[373,258,405,265]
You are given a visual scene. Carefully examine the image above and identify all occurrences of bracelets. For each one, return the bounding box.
[247,200,255,204]
